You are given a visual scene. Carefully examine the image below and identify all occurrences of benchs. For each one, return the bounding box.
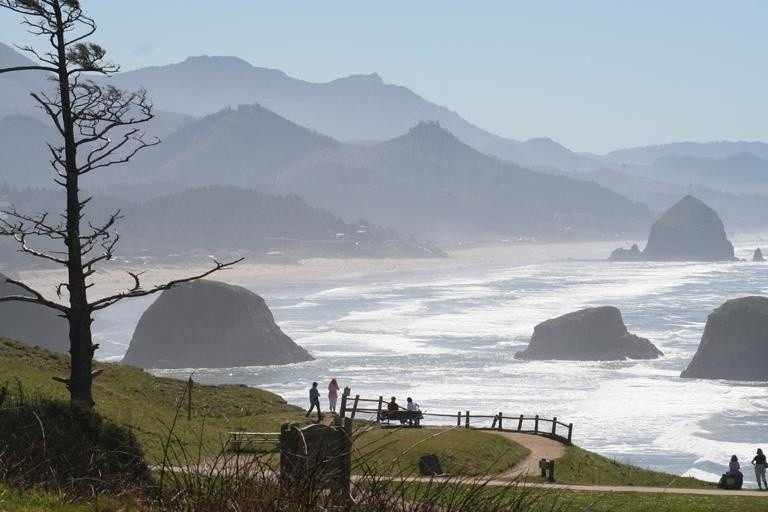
[378,409,423,429]
[228,430,281,452]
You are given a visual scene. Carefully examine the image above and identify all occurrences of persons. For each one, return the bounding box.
[305,382,323,421]
[327,379,340,414]
[406,396,420,427]
[729,454,743,491]
[387,396,398,412]
[750,447,768,491]
